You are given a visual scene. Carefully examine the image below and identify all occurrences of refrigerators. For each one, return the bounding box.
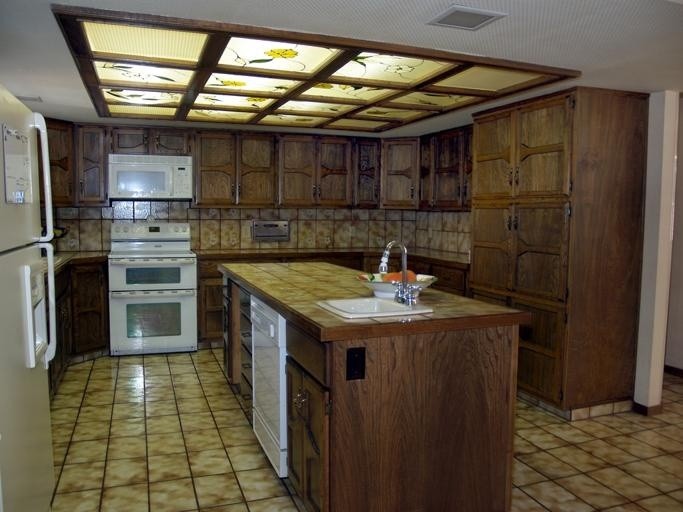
[0,88,56,512]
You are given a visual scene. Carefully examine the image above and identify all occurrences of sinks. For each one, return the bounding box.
[42,255,62,271]
[319,296,435,319]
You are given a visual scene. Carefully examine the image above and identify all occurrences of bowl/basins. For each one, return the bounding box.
[354,271,437,299]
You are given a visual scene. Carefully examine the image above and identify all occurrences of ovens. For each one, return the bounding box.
[107,257,198,356]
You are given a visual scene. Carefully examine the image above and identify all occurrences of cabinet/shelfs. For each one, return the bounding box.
[38,118,108,208]
[69,260,104,356]
[406,257,465,299]
[111,125,190,156]
[45,265,68,406]
[420,124,470,213]
[197,259,284,340]
[354,134,419,210]
[236,289,253,427]
[467,86,658,423]
[279,134,355,209]
[193,131,279,208]
[287,319,335,512]
[220,275,240,385]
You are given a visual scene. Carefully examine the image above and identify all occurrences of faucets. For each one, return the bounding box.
[379,238,409,305]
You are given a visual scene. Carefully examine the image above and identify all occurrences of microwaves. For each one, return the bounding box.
[106,153,193,201]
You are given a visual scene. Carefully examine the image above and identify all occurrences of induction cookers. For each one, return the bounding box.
[107,241,197,258]
